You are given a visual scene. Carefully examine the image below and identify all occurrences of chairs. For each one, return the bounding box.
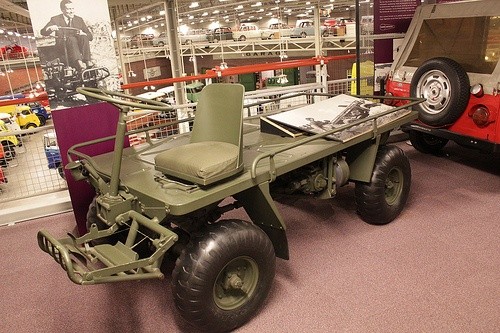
[152,80,244,188]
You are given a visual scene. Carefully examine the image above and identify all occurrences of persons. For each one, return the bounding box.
[41,0,95,69]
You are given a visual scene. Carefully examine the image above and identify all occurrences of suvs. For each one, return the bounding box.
[383,0,500,171]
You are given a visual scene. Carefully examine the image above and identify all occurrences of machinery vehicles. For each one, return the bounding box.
[37,80,428,333]
[38,28,109,106]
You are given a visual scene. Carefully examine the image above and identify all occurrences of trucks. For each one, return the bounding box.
[0,92,66,190]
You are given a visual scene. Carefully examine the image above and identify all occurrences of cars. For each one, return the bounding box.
[112,15,375,51]
[126,78,314,147]
